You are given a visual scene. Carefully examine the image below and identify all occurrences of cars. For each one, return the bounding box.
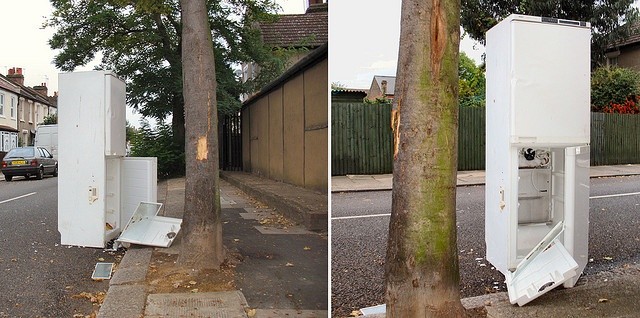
[2,147,57,182]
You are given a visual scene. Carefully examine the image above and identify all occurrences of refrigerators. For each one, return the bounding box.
[484,14,592,289]
[56,71,158,249]
